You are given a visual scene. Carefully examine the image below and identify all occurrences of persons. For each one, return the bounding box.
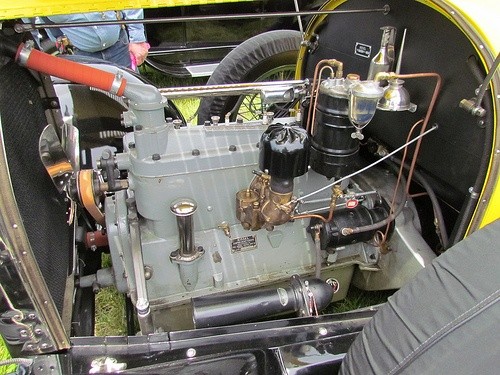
[38,8,150,70]
